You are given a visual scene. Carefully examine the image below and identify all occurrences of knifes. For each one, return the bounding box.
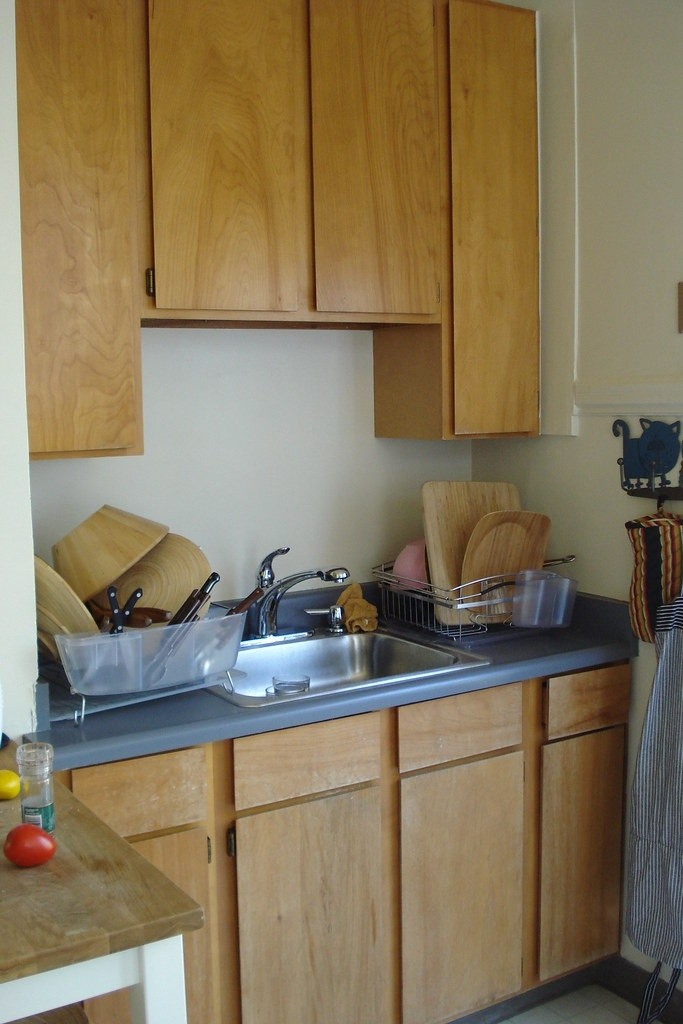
[78,586,142,687]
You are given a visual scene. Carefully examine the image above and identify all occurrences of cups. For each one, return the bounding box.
[264,685,305,698]
[272,673,311,692]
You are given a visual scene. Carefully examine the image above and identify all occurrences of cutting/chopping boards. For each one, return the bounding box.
[420,480,522,625]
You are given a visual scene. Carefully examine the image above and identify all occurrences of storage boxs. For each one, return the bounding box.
[52,612,248,697]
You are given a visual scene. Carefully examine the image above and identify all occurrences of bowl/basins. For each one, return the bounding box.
[391,536,429,591]
[34,555,101,660]
[54,601,248,695]
[49,503,169,603]
[512,569,578,628]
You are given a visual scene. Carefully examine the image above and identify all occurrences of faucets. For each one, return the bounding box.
[252,565,351,644]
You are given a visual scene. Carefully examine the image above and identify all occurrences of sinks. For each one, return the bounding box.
[203,624,496,708]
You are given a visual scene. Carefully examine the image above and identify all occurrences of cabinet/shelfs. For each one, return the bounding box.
[372,0,540,440]
[387,660,634,1024]
[134,0,442,331]
[15,0,144,461]
[53,708,396,1024]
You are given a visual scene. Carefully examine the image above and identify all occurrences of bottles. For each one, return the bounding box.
[17,742,54,840]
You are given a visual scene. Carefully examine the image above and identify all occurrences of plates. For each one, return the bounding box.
[459,512,551,615]
[86,532,212,622]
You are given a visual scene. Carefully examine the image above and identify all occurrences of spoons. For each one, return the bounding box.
[147,614,200,686]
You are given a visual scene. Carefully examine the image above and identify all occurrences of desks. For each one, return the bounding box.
[0,739,204,1024]
